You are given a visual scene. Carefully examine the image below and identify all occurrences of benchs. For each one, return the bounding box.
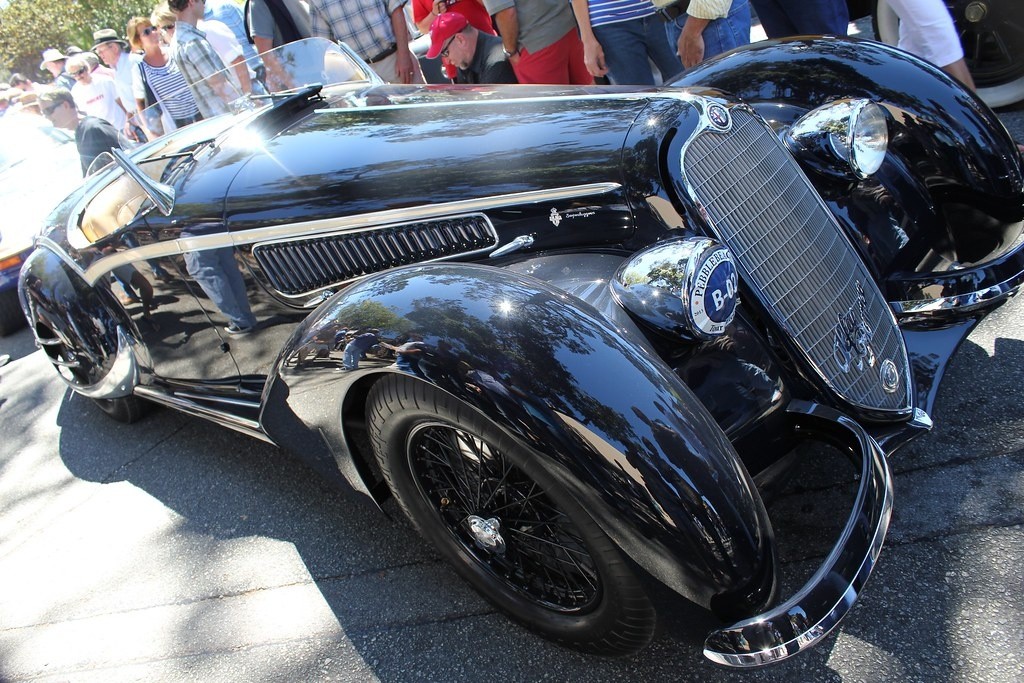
[81,117,232,241]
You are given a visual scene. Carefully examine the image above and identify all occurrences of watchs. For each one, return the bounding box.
[502,48,517,57]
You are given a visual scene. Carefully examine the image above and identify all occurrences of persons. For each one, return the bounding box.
[0,0,976,179]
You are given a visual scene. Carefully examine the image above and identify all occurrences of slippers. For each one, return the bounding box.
[140,316,160,333]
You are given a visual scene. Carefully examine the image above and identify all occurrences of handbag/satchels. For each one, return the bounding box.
[139,62,163,118]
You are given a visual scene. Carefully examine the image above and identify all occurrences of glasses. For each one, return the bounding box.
[160,25,174,31]
[441,30,462,57]
[42,102,62,116]
[69,66,85,76]
[141,26,157,37]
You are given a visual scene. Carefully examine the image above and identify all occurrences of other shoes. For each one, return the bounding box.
[335,367,349,372]
[225,323,253,333]
[123,297,139,305]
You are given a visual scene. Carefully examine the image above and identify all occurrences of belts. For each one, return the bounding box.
[364,43,397,64]
[655,0,690,23]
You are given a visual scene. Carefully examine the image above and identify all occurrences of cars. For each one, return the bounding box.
[15,34,1024,668]
[0,112,86,338]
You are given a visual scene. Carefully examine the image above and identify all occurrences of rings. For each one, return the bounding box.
[409,71,414,74]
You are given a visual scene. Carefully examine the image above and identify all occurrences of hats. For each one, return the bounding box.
[91,29,128,51]
[40,49,68,70]
[21,93,39,109]
[426,12,469,59]
